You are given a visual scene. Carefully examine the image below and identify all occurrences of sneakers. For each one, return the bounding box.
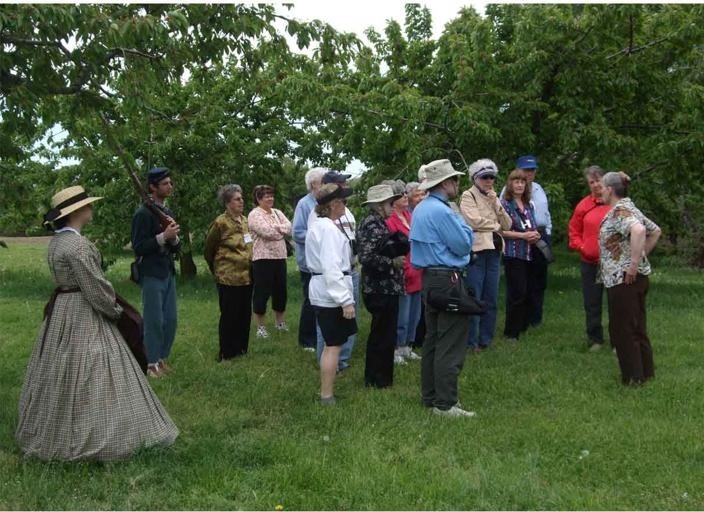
[274,320,289,332]
[427,397,478,419]
[254,324,271,338]
[391,347,422,366]
[147,362,169,380]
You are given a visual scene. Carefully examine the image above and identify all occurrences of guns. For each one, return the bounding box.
[114,145,175,231]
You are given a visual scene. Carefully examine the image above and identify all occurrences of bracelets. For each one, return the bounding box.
[629,267,637,272]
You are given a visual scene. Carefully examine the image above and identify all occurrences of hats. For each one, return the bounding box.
[148,166,171,184]
[467,157,499,181]
[381,178,422,197]
[43,183,104,226]
[320,171,353,184]
[416,157,466,191]
[517,154,539,170]
[316,184,355,204]
[360,184,403,207]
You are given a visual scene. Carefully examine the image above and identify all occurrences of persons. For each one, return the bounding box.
[357,155,552,420]
[247,184,292,340]
[131,167,181,379]
[568,165,618,356]
[203,184,254,364]
[596,170,662,388]
[14,184,181,465]
[292,167,358,404]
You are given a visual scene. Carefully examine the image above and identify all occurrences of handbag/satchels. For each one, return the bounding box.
[530,235,556,267]
